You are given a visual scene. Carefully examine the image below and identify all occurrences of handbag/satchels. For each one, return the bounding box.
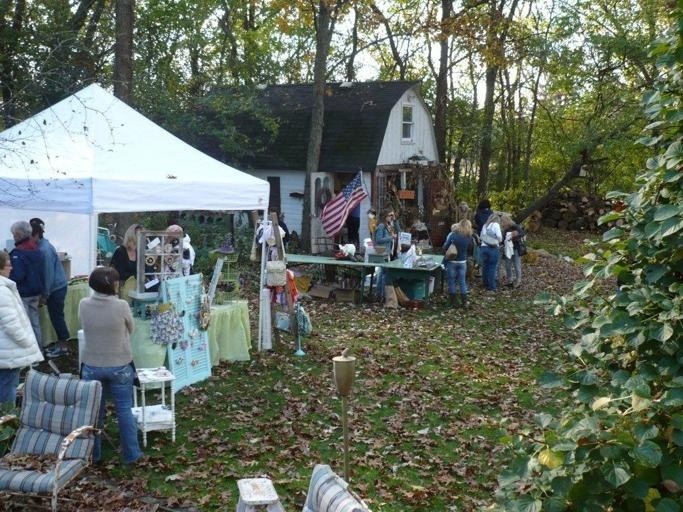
[198,273,210,330]
[518,235,526,256]
[250,220,312,337]
[150,280,183,345]
[445,231,457,261]
[480,222,499,248]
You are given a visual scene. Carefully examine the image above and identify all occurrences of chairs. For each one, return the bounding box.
[0,366,104,512]
[301,464,376,512]
[97,226,119,258]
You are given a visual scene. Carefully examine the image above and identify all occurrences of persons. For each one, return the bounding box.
[109,223,160,306]
[162,225,190,280]
[1,249,45,407]
[30,218,71,357]
[76,266,143,465]
[9,220,46,368]
[268,206,289,253]
[347,199,527,309]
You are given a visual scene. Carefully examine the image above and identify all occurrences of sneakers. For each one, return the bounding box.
[45,344,68,358]
[44,342,58,353]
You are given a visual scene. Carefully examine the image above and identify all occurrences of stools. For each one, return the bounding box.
[236,478,285,512]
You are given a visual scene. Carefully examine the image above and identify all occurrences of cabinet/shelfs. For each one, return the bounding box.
[216,248,242,300]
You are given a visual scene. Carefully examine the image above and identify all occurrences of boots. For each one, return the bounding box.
[450,294,455,304]
[461,294,465,303]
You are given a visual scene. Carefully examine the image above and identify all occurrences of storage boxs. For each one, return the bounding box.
[335,288,361,305]
[425,275,435,295]
[398,278,426,299]
[308,284,334,301]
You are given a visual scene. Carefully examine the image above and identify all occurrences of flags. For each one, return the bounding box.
[319,170,368,241]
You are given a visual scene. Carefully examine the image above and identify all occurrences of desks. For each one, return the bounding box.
[77,298,249,392]
[38,278,95,348]
[130,367,177,448]
[286,253,446,307]
